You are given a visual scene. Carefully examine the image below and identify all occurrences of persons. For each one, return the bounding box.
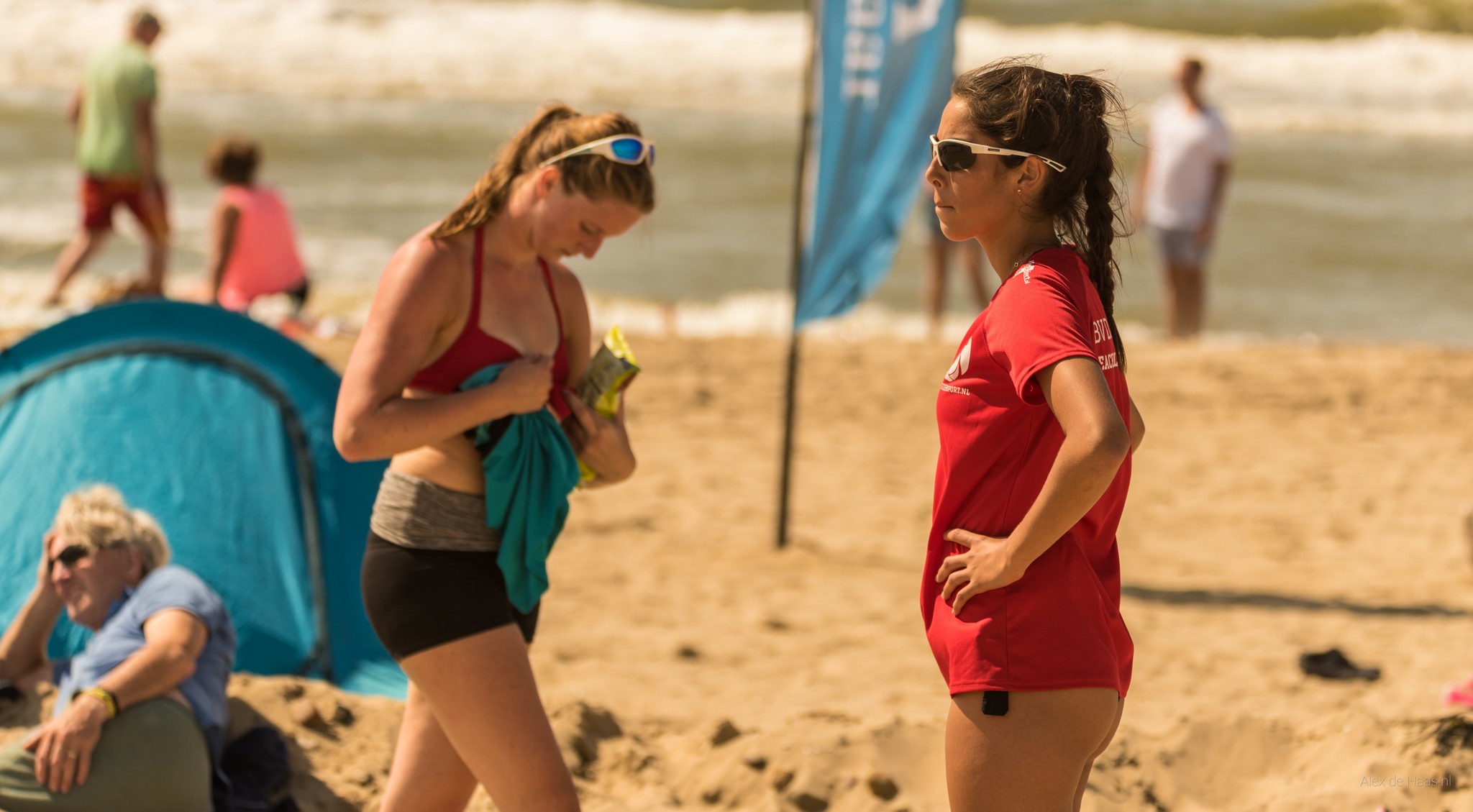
[44,10,170,306]
[919,185,989,346]
[332,104,660,812]
[203,137,310,318]
[0,485,232,812]
[919,59,1145,812]
[1127,55,1232,340]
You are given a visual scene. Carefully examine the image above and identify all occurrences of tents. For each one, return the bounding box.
[0,298,408,701]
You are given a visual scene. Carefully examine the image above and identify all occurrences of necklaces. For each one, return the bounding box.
[1009,255,1033,276]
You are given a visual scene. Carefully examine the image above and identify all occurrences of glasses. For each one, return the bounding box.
[536,134,660,170]
[929,133,1067,175]
[45,540,122,574]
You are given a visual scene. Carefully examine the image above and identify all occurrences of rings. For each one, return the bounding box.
[66,750,77,759]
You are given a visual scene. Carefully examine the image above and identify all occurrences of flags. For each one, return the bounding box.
[794,1,963,330]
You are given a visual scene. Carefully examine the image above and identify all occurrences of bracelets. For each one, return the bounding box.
[68,687,119,719]
